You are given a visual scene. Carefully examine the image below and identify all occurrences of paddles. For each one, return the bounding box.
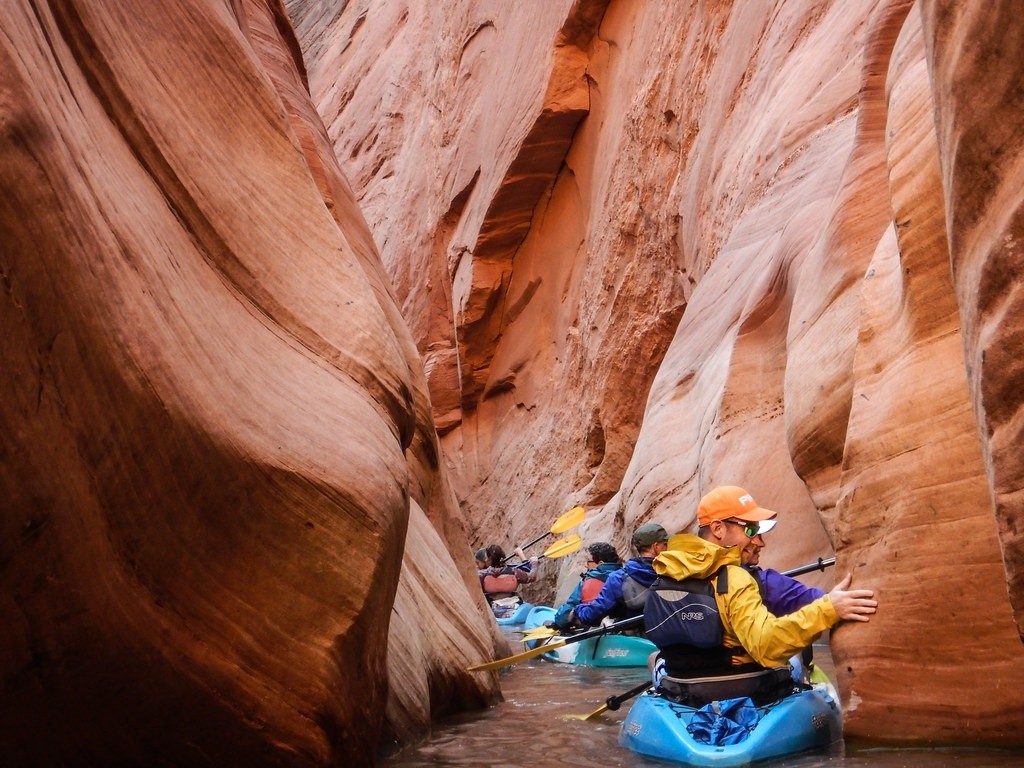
[465,556,836,672]
[503,506,586,563]
[583,679,655,723]
[510,624,589,642]
[515,534,583,570]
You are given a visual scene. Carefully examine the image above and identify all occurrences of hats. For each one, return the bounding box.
[757,520,777,535]
[633,523,672,548]
[697,485,777,527]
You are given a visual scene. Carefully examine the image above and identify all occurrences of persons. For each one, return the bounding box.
[475,547,531,572]
[555,542,623,633]
[570,523,669,637]
[741,520,828,666]
[479,545,538,618]
[643,486,879,690]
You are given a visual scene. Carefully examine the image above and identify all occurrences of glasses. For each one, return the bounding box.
[587,558,594,565]
[720,520,760,538]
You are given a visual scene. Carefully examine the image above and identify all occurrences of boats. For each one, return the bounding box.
[524,606,659,667]
[496,600,535,626]
[618,664,843,768]
